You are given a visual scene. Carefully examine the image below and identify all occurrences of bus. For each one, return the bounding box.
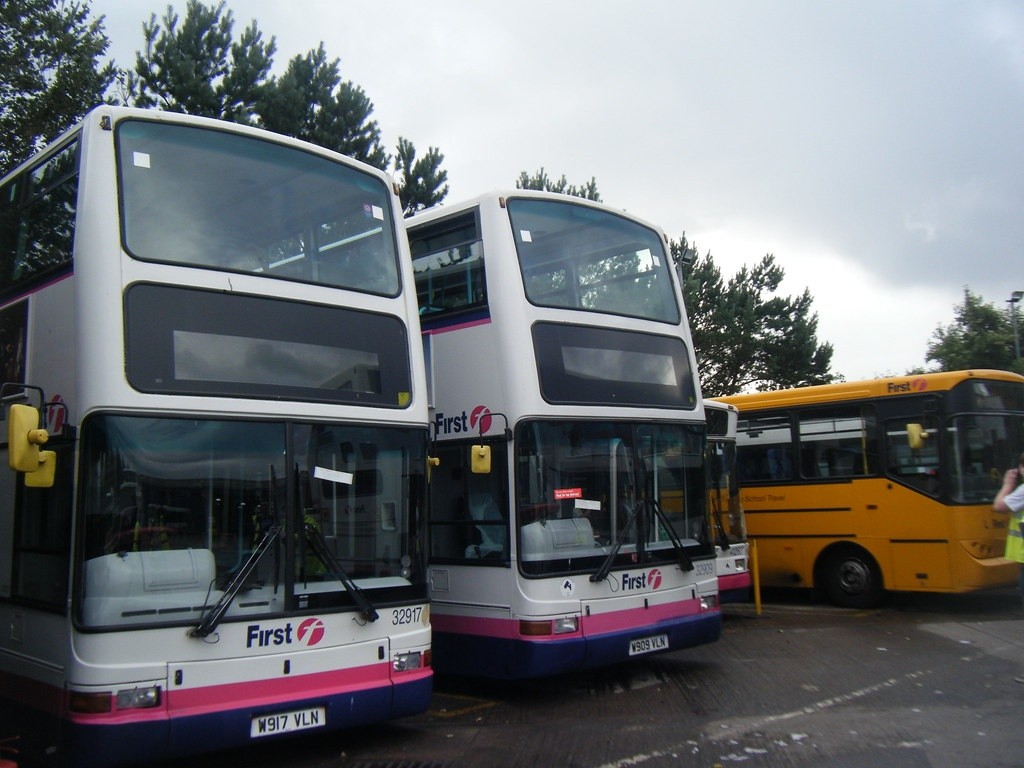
[700,401,752,606]
[1,103,436,768]
[256,189,722,686]
[705,368,1023,610]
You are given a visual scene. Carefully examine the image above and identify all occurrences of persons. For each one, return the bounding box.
[992,451,1024,592]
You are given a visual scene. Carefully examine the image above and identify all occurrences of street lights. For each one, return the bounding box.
[1009,291,1024,375]
